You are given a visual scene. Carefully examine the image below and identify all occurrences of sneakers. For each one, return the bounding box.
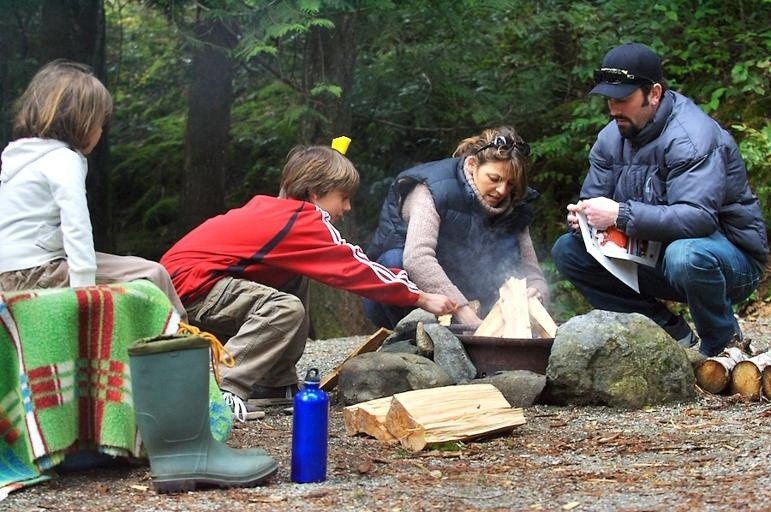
[248,380,305,407]
[663,316,699,349]
[220,389,266,421]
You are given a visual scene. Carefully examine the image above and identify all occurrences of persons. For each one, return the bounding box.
[360,123,550,338]
[549,39,770,358]
[159,142,459,423]
[0,55,191,326]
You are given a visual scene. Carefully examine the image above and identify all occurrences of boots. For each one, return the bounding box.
[126,332,280,494]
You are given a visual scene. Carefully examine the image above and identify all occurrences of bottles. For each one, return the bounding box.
[290,367,329,484]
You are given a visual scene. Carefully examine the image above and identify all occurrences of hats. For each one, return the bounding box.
[588,41,662,99]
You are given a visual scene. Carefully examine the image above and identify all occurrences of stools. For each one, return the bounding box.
[0,280,234,500]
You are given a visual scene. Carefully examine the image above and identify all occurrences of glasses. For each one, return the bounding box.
[475,135,530,156]
